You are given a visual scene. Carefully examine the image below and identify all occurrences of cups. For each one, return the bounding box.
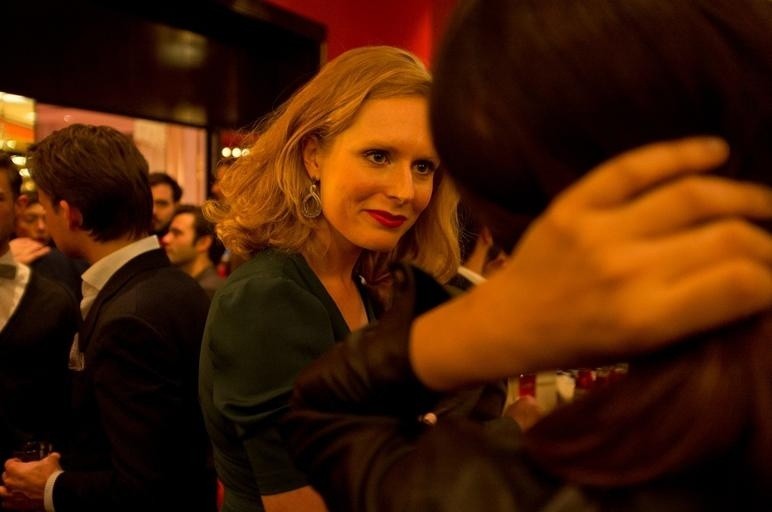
[18,441,52,463]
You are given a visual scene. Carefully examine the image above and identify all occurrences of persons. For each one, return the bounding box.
[422,0,772,512]
[195,43,772,511]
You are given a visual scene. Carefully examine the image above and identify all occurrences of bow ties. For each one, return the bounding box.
[0,264,17,279]
